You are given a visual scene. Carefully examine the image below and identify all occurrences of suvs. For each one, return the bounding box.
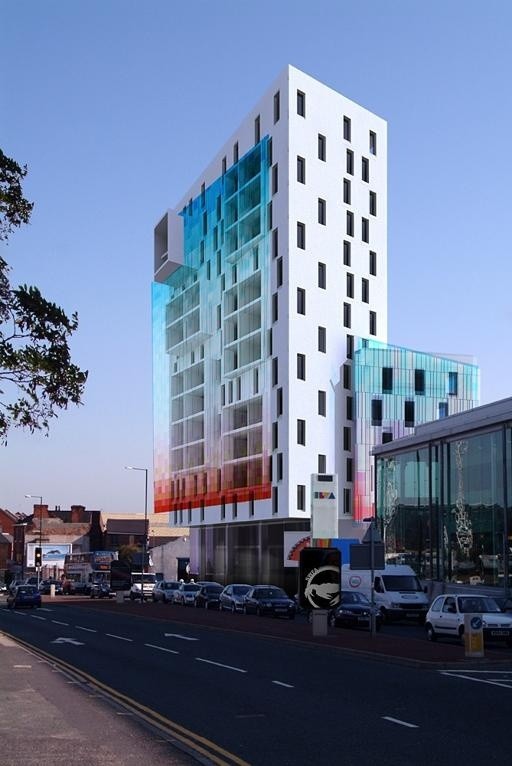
[90,570,116,600]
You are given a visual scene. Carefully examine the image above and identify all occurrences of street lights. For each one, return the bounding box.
[360,515,378,638]
[125,465,147,603]
[25,494,43,548]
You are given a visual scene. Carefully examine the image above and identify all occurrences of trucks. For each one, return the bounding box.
[337,564,430,619]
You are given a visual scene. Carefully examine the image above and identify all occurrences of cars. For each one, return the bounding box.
[329,589,385,630]
[423,591,512,643]
[0,570,112,610]
[396,547,512,586]
[131,571,298,621]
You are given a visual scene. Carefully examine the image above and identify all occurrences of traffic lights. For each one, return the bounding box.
[35,548,41,567]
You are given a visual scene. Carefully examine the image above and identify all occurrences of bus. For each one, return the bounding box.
[62,551,121,595]
